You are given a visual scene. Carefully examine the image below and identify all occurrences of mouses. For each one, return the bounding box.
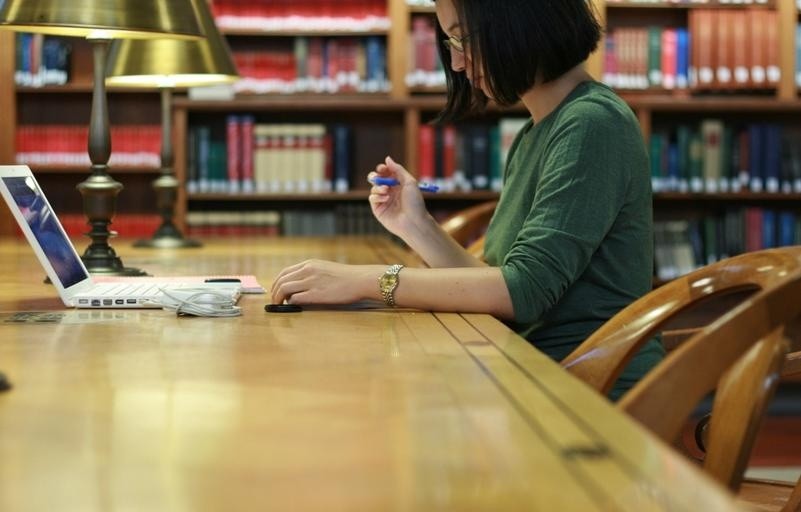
[175,291,234,316]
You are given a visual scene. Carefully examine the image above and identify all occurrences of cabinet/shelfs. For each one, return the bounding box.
[1,2,800,284]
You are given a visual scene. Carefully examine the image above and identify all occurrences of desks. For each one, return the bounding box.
[2,239,756,512]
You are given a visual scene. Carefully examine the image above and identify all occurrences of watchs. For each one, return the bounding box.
[377,264,406,307]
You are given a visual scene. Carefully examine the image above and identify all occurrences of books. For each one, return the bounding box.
[403,108,532,192]
[653,203,801,281]
[189,0,452,94]
[188,114,351,193]
[648,108,801,193]
[603,5,781,94]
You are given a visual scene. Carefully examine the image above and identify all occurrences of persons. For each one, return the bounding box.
[271,0,668,402]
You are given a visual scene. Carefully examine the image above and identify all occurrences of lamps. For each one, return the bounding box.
[105,2,238,248]
[0,0,208,285]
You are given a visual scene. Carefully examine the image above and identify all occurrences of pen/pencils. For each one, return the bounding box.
[367,176,440,193]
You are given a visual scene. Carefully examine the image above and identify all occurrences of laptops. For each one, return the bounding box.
[0,164,242,309]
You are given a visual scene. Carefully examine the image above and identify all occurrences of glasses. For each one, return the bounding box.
[444,29,471,52]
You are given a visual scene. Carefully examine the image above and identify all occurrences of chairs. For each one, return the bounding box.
[556,249,799,509]
